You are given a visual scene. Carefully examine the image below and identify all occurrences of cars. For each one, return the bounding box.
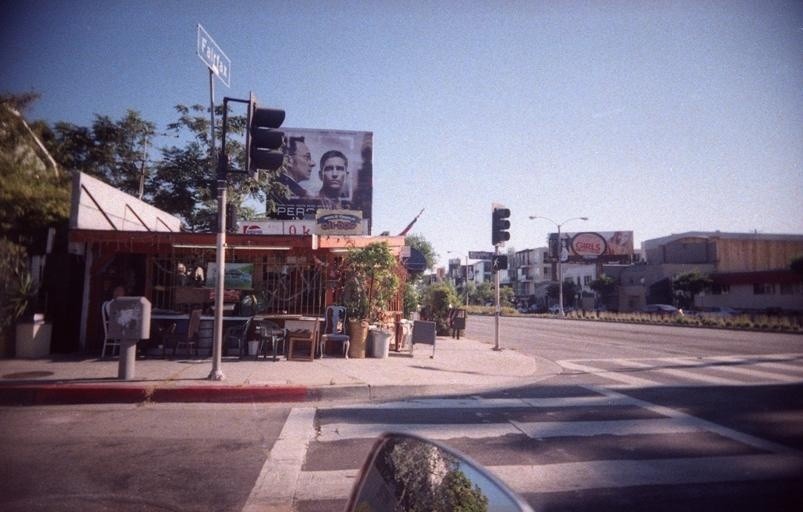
[634,304,685,320]
[548,304,575,314]
[517,304,539,313]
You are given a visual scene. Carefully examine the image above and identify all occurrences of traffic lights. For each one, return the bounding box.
[247,90,285,177]
[492,207,510,247]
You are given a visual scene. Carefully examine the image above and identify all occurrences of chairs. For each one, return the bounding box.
[99,301,122,359]
[220,315,320,361]
[170,308,203,357]
[321,304,350,361]
[407,319,438,359]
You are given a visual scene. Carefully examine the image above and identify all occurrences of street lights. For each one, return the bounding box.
[529,216,588,317]
[447,250,468,306]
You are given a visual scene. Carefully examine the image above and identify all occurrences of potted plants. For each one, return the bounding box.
[341,239,400,359]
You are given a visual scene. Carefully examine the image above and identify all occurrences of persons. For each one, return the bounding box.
[606,232,633,255]
[270,135,314,198]
[314,150,348,198]
[106,287,176,345]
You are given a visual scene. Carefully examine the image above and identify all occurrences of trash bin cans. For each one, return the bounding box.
[372,332,392,358]
